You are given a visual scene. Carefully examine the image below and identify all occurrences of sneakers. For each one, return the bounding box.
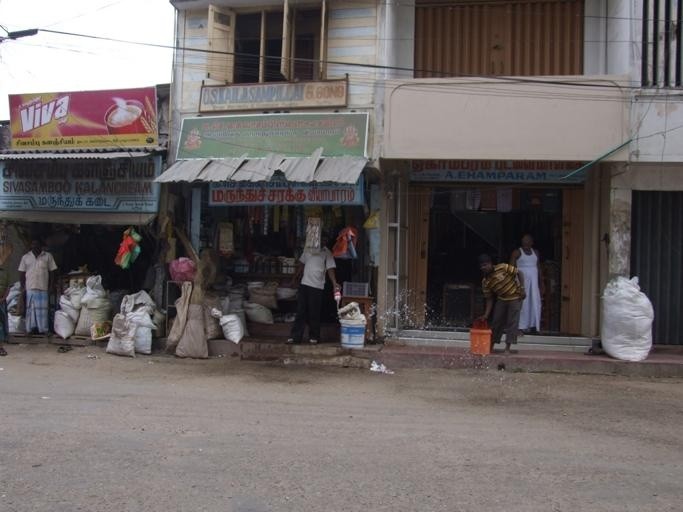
[285,338,299,344]
[308,337,320,345]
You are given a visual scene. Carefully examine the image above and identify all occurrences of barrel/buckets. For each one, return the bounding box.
[470,328,492,356]
[340,319,365,348]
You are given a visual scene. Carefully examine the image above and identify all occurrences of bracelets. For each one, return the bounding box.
[519,286,525,290]
[295,272,299,277]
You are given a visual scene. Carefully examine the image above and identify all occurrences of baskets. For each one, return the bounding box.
[342,282,368,297]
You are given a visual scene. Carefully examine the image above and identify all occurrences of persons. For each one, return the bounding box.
[16,236,58,335]
[477,254,525,357]
[285,233,340,345]
[0,264,10,356]
[508,235,545,334]
